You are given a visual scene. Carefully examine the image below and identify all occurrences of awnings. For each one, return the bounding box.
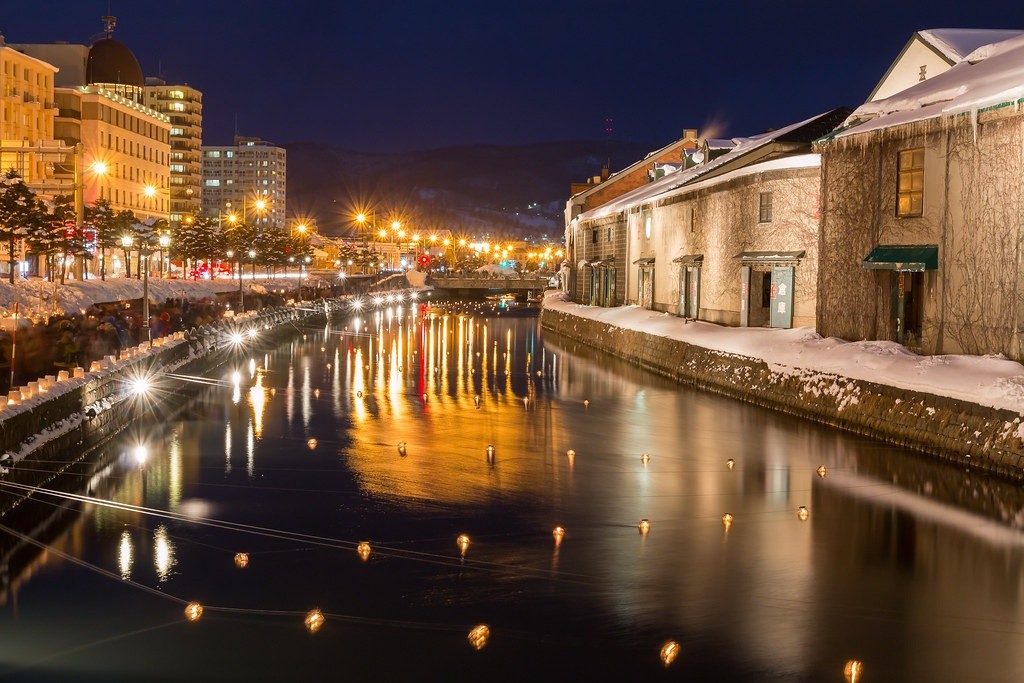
[862,244,938,271]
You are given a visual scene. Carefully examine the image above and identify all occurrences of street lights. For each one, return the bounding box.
[413,234,419,261]
[356,214,364,242]
